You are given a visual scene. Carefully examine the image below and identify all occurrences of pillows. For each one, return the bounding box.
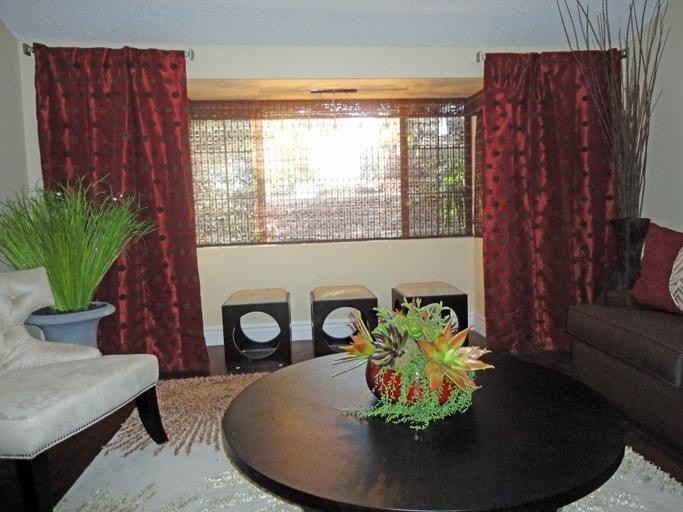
[626,221,681,314]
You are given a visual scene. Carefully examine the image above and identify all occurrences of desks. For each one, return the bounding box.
[221,351,627,511]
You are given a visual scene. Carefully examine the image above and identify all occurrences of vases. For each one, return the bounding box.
[365,358,457,406]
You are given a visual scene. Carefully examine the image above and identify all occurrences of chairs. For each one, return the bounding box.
[0,266,169,512]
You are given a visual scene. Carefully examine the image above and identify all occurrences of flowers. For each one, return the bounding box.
[323,293,496,432]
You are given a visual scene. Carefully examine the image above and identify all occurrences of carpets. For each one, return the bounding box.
[51,369,683,511]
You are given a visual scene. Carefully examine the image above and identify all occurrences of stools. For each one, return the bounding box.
[221,281,468,375]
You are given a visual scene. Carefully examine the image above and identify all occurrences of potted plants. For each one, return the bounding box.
[555,1,670,293]
[0,171,161,348]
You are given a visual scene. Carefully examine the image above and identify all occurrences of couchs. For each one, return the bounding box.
[558,290,683,459]
